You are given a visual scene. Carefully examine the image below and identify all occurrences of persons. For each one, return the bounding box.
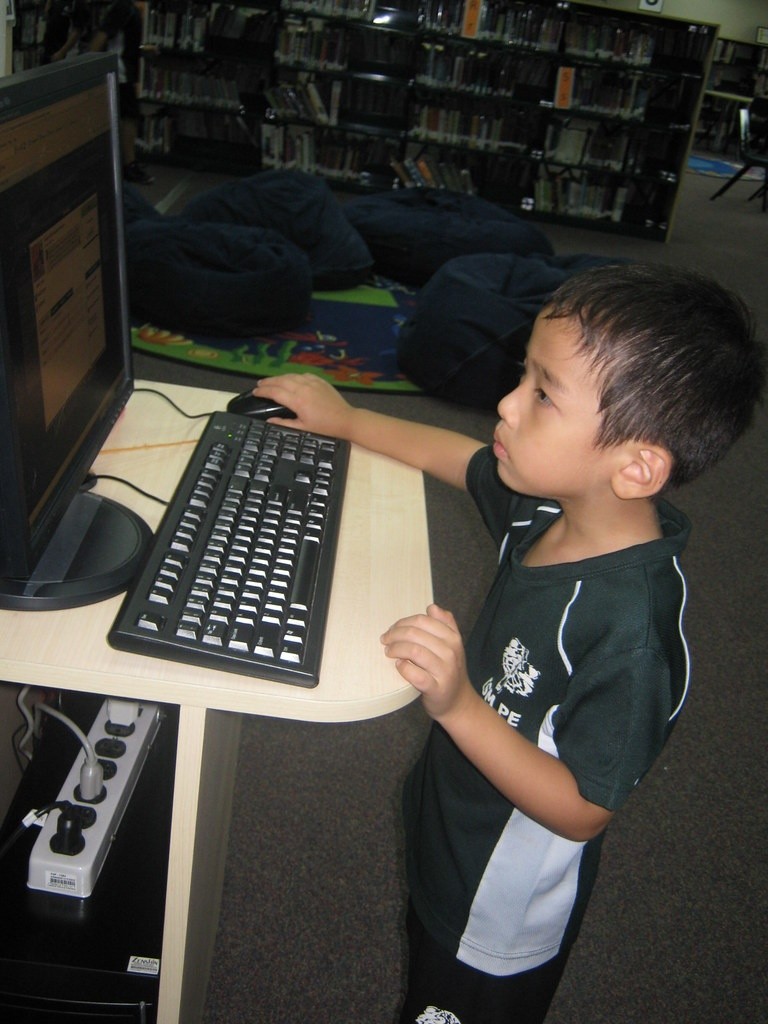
[89,3,153,184]
[253,261,768,1023]
[41,0,84,64]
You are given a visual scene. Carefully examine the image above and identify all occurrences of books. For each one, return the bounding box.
[14,6,44,74]
[146,3,767,225]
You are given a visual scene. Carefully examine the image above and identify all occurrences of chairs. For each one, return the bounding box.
[710,95,768,210]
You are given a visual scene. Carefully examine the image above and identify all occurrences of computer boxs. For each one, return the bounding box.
[0,690,181,1023]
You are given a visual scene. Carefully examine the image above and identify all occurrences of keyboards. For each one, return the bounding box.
[107,408,352,690]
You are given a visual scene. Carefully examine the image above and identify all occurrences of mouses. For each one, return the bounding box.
[227,385,296,421]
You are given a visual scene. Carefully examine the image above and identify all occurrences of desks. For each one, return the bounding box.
[1,373,439,1024]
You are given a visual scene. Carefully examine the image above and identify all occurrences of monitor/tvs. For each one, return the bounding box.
[2,49,133,582]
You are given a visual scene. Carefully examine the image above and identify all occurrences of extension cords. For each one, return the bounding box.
[26,695,167,897]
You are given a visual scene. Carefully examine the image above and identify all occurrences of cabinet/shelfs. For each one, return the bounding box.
[690,35,768,145]
[11,0,719,244]
